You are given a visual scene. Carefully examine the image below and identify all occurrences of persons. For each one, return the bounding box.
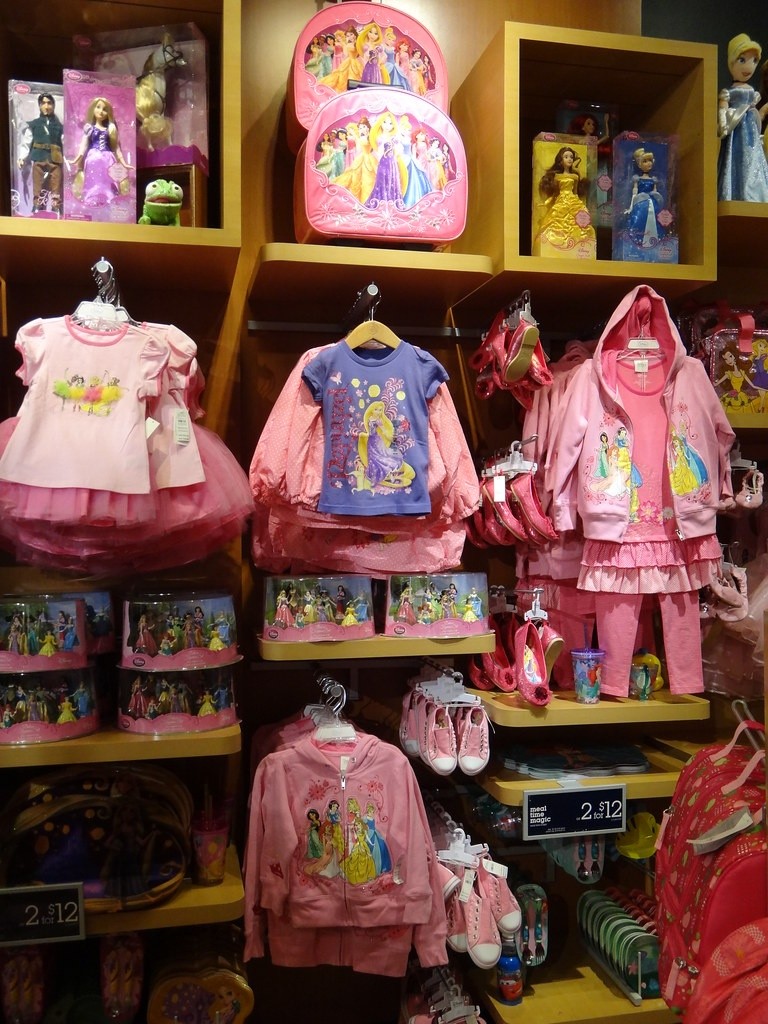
[16,92,63,215]
[533,146,596,258]
[570,112,609,209]
[1,573,490,745]
[718,34,767,202]
[67,97,134,214]
[623,148,665,249]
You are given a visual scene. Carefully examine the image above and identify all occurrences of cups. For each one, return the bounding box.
[632,664,659,701]
[572,649,605,705]
[193,819,229,885]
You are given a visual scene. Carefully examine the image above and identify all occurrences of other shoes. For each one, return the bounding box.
[471,612,564,711]
[399,689,490,776]
[465,474,559,548]
[440,855,521,969]
[467,312,555,411]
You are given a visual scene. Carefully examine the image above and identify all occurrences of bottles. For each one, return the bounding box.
[497,934,524,1005]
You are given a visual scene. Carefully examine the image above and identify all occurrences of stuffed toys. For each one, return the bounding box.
[139,178,184,227]
